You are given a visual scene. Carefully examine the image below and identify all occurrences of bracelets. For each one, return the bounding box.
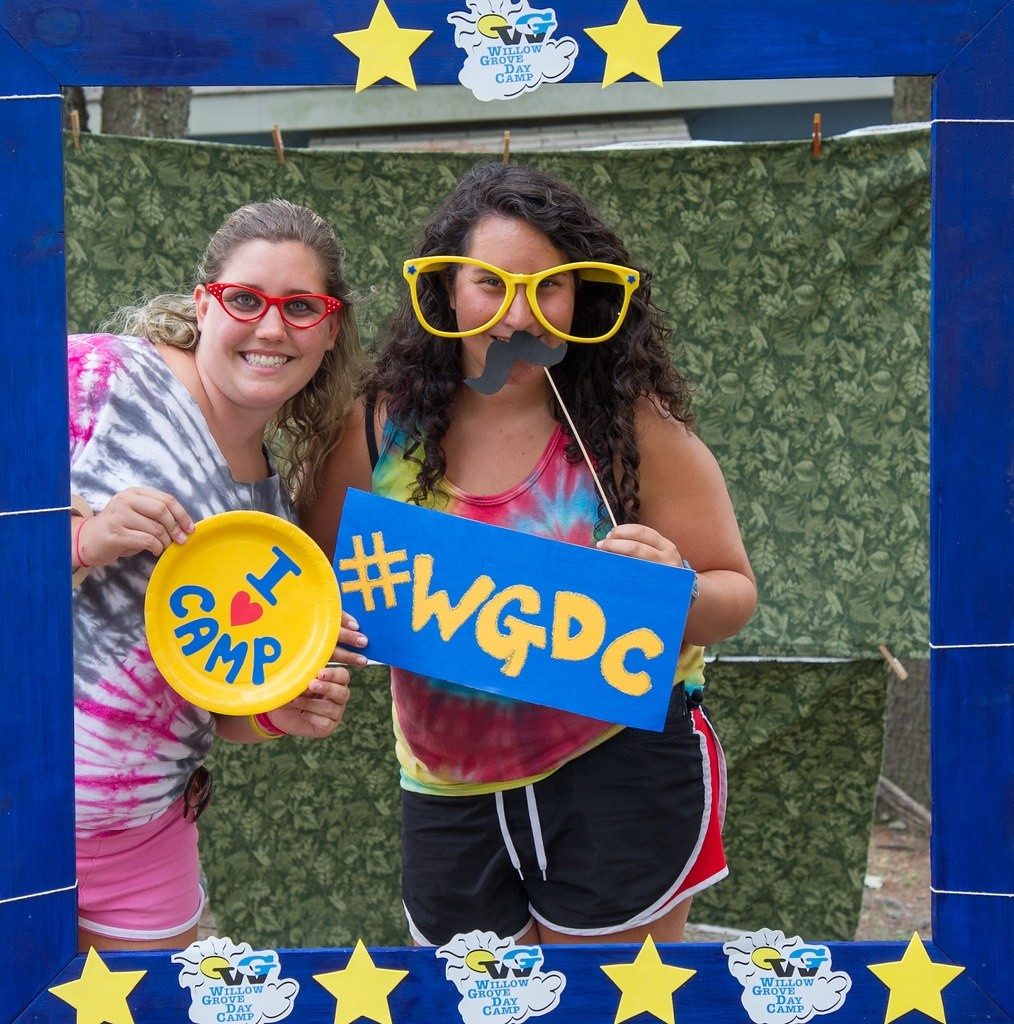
[76,519,89,567]
[682,559,699,606]
[250,713,287,739]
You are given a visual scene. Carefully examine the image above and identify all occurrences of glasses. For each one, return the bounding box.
[204,282,346,330]
[402,255,641,344]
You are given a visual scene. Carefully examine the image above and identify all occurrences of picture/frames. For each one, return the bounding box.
[0,0,1014,1024]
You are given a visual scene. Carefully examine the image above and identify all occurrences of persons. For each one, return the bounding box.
[292,165,758,949]
[68,196,352,957]
